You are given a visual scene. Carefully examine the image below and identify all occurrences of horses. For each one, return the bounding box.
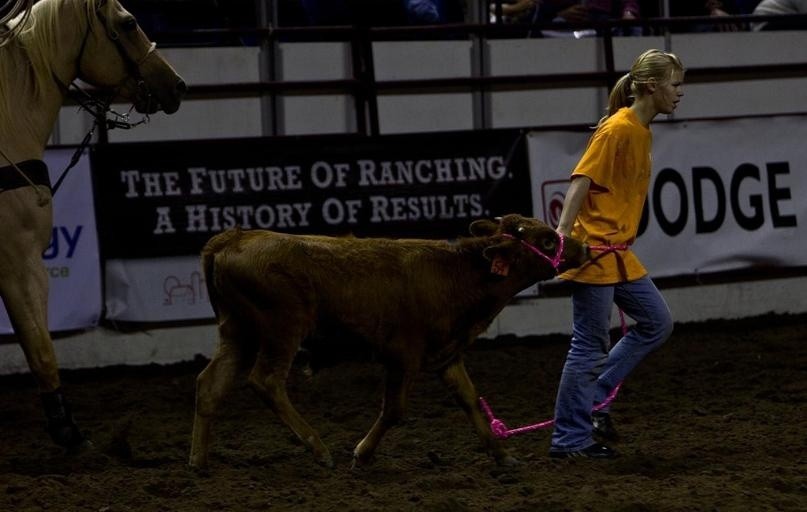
[0,1,188,475]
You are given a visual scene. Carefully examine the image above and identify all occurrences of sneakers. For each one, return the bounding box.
[547,443,621,463]
[593,410,621,443]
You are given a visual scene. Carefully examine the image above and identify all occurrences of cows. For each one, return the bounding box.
[188,212,590,479]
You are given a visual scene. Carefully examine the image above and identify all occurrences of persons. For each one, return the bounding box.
[547,49,686,460]
